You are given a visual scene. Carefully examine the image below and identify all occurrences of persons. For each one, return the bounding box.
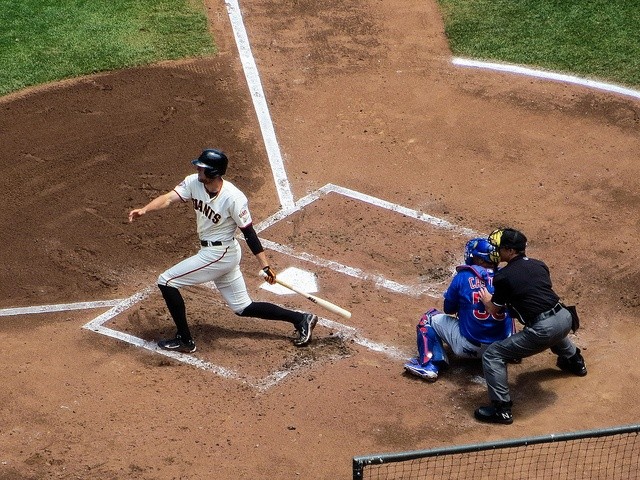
[474,227,587,425]
[404,237,516,382]
[128,149,318,353]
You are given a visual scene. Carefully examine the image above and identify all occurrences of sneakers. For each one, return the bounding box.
[292,312,318,348]
[474,399,513,424]
[404,356,439,382]
[157,333,197,353]
[556,347,587,376]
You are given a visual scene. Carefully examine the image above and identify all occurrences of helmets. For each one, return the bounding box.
[464,236,500,266]
[487,225,527,267]
[191,150,228,179]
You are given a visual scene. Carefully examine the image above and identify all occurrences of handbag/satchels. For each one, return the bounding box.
[557,301,579,334]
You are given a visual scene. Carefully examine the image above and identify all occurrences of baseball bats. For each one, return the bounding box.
[259,269,351,320]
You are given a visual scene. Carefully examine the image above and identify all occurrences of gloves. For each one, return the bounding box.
[263,266,277,285]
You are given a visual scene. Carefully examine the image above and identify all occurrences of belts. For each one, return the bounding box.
[201,237,234,247]
[467,339,481,348]
[544,304,562,319]
